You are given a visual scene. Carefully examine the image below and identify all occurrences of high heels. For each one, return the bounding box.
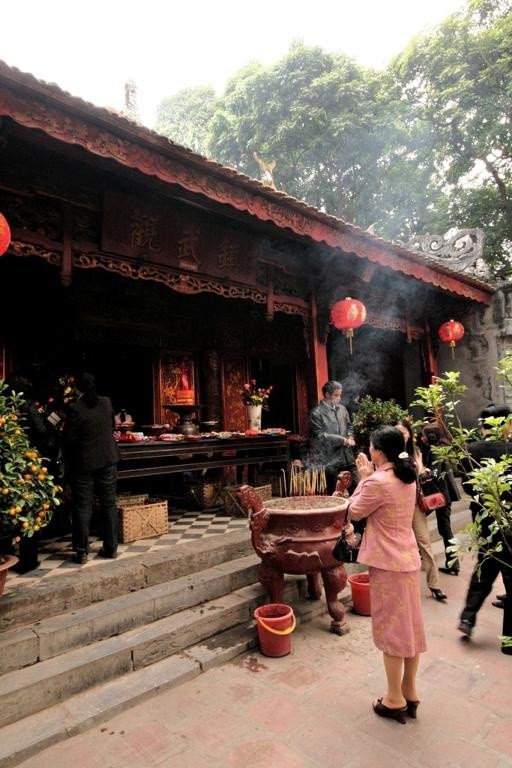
[373,697,419,723]
[439,567,459,575]
[429,588,446,600]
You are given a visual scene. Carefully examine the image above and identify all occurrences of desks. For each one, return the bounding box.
[115,431,294,493]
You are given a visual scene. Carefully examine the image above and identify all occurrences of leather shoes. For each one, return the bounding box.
[492,594,506,608]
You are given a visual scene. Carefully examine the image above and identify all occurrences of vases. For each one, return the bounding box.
[0,553,20,599]
[247,405,262,430]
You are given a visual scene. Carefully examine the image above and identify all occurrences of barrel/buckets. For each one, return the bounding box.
[254,603,297,657]
[348,573,370,616]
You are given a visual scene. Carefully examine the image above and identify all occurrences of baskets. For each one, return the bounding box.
[115,494,168,543]
[190,481,272,515]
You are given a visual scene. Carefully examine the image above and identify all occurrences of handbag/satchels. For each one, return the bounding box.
[333,503,359,562]
[419,493,446,512]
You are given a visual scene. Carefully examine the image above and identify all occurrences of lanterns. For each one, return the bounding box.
[439,318,465,362]
[330,296,368,356]
[0,212,12,257]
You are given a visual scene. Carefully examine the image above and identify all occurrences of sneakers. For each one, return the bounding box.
[460,619,471,635]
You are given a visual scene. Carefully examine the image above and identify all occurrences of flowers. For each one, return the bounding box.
[0,377,65,544]
[241,377,273,403]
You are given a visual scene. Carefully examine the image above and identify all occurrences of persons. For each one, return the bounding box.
[59,371,122,565]
